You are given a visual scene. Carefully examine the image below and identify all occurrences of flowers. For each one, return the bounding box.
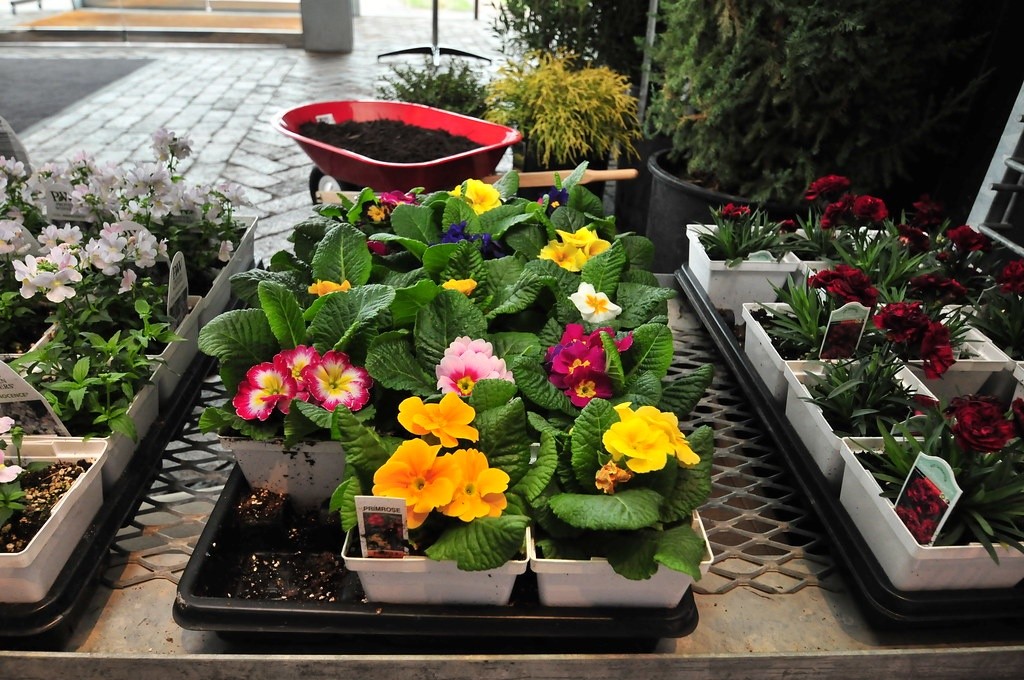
[0,126,253,531]
[191,160,714,583]
[689,175,1024,567]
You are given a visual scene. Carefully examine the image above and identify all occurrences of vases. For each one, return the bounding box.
[743,303,902,405]
[529,507,715,610]
[1009,363,1024,411]
[0,293,79,358]
[791,230,889,291]
[878,303,1007,411]
[342,526,530,604]
[30,295,201,404]
[783,359,955,500]
[687,225,801,326]
[1,357,166,492]
[200,215,260,328]
[840,436,1024,592]
[1,440,110,604]
[802,262,843,309]
[213,427,396,516]
[948,305,1023,411]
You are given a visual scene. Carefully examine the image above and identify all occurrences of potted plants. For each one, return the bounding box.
[482,0,997,274]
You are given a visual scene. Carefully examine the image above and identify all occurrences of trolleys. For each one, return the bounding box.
[270,96,640,211]
